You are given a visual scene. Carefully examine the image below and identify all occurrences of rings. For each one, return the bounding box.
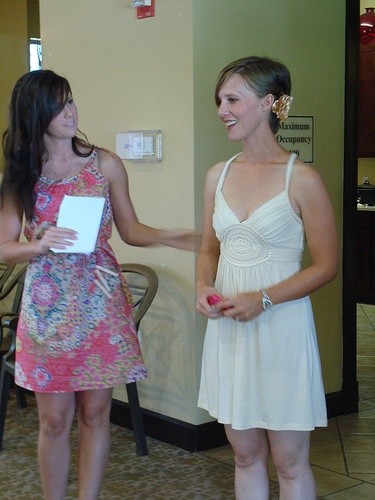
[236,315,240,322]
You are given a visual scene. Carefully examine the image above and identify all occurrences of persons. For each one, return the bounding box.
[193,54,337,500]
[0,69,201,500]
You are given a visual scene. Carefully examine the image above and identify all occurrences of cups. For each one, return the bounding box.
[356,180,375,206]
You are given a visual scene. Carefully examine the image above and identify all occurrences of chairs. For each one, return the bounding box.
[0,261,16,291]
[0,264,44,408]
[0,262,159,457]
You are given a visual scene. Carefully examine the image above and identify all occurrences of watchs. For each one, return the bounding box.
[259,289,274,312]
